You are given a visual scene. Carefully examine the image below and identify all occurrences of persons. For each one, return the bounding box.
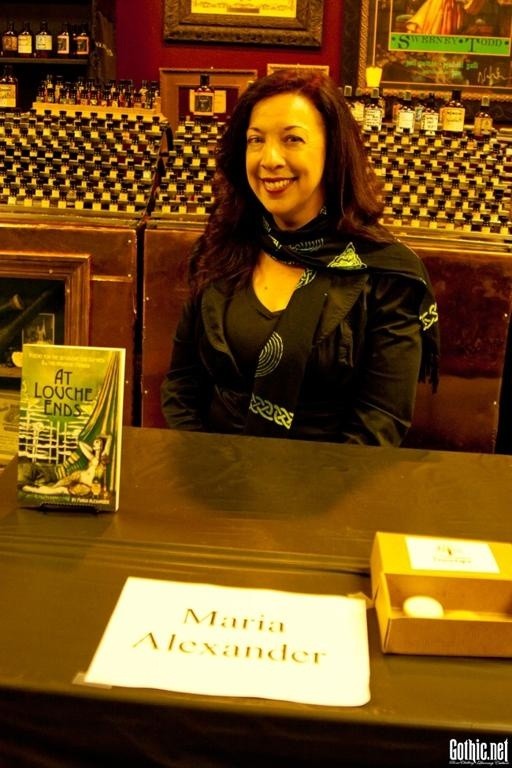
[160,71,441,447]
[22,433,114,495]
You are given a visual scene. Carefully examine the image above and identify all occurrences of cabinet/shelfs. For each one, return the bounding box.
[0,1,115,87]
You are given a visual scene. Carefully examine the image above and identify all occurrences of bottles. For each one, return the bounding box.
[36,74,157,106]
[354,87,365,121]
[344,87,356,120]
[194,74,215,116]
[160,114,230,219]
[384,91,512,139]
[56,21,70,56]
[18,21,34,57]
[372,86,386,121]
[360,127,511,237]
[0,65,19,108]
[72,25,80,57]
[76,24,89,56]
[1,19,17,56]
[363,90,371,101]
[366,99,381,133]
[0,109,159,215]
[35,21,53,57]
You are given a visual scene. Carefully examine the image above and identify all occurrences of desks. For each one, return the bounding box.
[0,427,512,730]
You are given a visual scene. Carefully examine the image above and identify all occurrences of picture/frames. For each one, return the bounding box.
[158,68,260,136]
[267,63,330,78]
[356,0,512,102]
[0,250,93,377]
[162,0,319,45]
